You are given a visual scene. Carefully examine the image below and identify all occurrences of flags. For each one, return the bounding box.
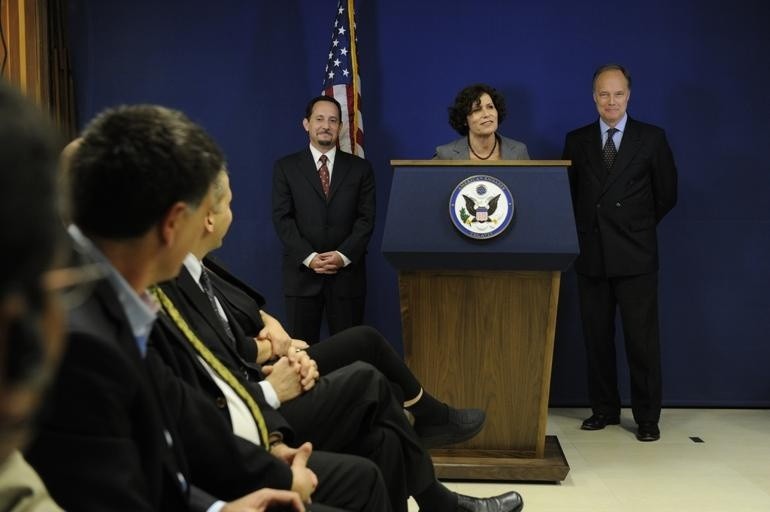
[319,0,367,161]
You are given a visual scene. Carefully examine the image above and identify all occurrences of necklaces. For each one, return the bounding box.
[467,139,499,160]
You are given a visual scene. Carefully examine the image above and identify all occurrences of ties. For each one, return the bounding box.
[199,265,266,381]
[602,128,618,173]
[317,155,330,200]
[155,288,271,453]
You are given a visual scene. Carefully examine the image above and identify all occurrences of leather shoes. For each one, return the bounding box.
[637,422,660,441]
[457,491,524,512]
[582,413,621,430]
[413,403,487,449]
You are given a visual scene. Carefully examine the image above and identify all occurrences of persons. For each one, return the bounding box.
[2,78,74,512]
[267,96,381,346]
[555,62,682,445]
[46,136,389,512]
[9,97,310,512]
[143,128,525,511]
[426,83,534,166]
[203,254,489,452]
